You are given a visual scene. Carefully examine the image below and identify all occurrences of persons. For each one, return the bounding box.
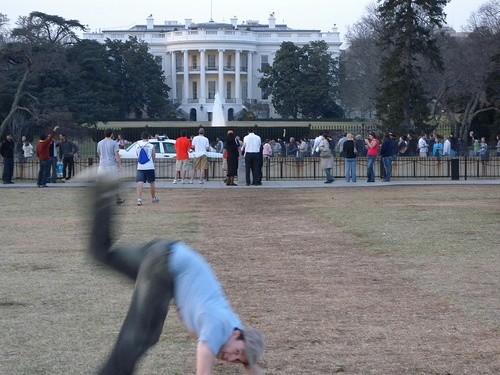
[240,127,261,185]
[319,133,335,183]
[381,135,394,183]
[37,125,59,187]
[173,131,192,184]
[136,131,160,205]
[418,132,428,157]
[343,133,357,182]
[215,136,223,153]
[96,129,125,204]
[189,128,210,184]
[59,134,78,182]
[0,133,16,184]
[115,134,124,149]
[17,135,33,179]
[224,130,240,186]
[68,165,269,375]
[364,132,379,182]
[235,131,500,158]
[47,133,58,183]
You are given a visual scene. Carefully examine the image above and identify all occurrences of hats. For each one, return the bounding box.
[199,128,204,134]
[228,130,233,134]
[243,327,263,370]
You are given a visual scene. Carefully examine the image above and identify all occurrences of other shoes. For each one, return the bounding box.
[151,198,160,203]
[136,201,142,205]
[181,180,185,184]
[199,180,203,184]
[174,180,177,183]
[324,180,335,183]
[2,180,15,184]
[37,184,48,187]
[227,182,237,186]
[189,180,193,183]
[61,178,66,182]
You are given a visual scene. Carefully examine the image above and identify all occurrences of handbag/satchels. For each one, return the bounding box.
[320,148,333,158]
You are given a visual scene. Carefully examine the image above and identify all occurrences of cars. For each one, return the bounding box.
[118,137,223,164]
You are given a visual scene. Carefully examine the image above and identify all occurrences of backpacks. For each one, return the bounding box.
[138,143,151,164]
